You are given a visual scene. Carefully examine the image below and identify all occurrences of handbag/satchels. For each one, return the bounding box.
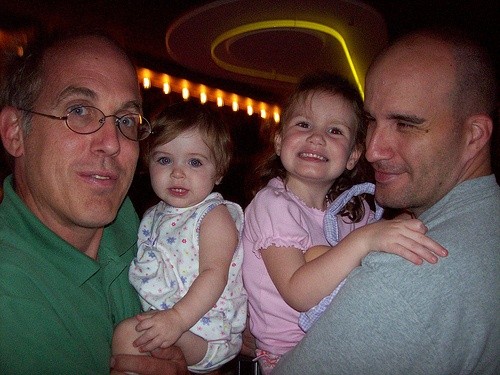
[297,182,384,335]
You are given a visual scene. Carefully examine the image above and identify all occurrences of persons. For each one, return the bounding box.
[243,72,451,375]
[0,20,191,375]
[113,103,248,375]
[242,25,500,375]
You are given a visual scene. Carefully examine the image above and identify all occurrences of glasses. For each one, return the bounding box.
[10,105,155,142]
